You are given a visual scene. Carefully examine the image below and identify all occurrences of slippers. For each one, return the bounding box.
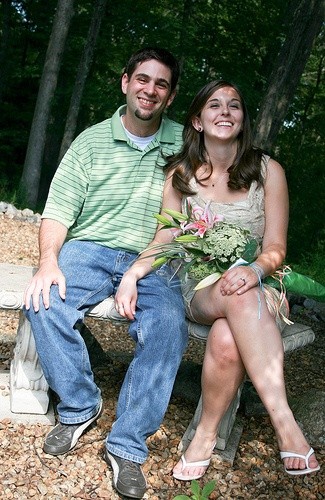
[278,447,320,476]
[172,437,219,481]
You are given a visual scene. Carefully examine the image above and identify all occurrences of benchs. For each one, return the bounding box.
[0,263,315,450]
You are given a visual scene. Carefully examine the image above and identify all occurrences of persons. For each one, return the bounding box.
[111,79,321,483]
[19,47,189,499]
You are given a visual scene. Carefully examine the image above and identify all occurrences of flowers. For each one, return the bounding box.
[136,196,253,281]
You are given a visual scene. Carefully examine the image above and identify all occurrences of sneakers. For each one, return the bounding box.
[101,449,146,499]
[43,398,103,455]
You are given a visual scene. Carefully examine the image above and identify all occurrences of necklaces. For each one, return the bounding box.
[198,164,229,188]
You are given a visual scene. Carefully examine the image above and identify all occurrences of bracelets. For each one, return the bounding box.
[248,262,266,283]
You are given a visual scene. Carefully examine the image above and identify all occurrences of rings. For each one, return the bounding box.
[239,277,246,283]
